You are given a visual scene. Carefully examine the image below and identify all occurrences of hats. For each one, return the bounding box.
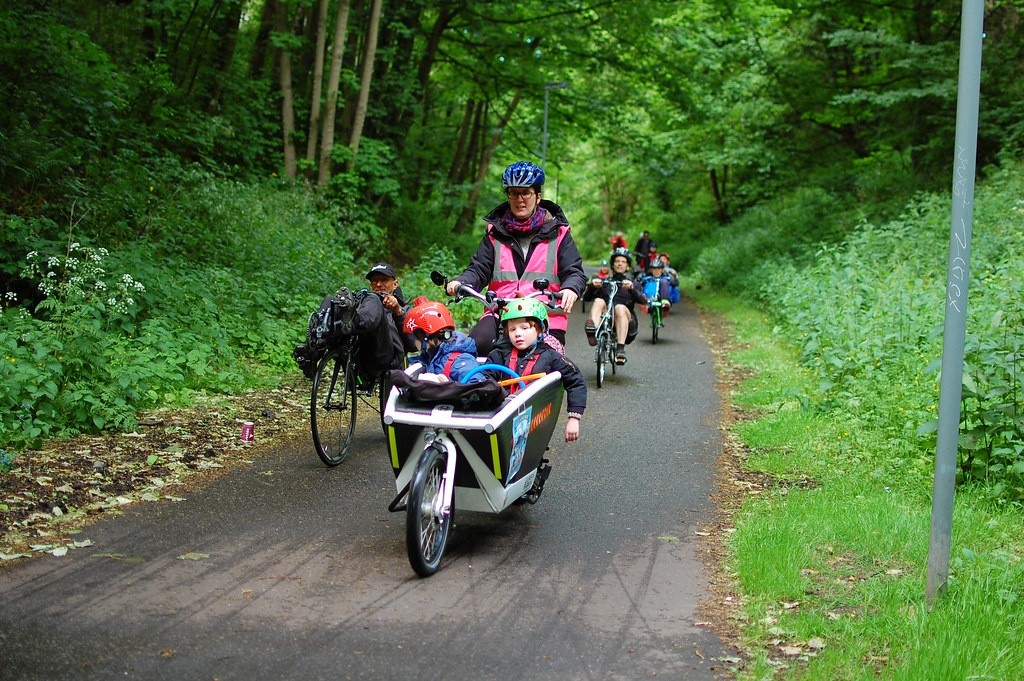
[365,262,395,282]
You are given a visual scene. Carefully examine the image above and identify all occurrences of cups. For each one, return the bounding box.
[241,422,254,442]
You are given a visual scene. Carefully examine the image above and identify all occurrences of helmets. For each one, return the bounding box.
[500,291,549,335]
[649,258,665,268]
[610,246,632,267]
[501,161,545,189]
[650,243,658,248]
[403,296,455,335]
[601,260,609,266]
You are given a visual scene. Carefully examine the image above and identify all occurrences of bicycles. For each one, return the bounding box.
[378,269,572,577]
[586,280,631,389]
[637,275,672,343]
[310,287,398,468]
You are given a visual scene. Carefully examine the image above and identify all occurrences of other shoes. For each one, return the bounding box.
[662,301,670,317]
[294,344,319,379]
[614,352,626,365]
[353,358,370,395]
[640,303,648,315]
[584,319,598,346]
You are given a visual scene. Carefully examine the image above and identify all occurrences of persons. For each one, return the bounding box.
[633,258,677,317]
[446,159,589,357]
[403,301,487,385]
[659,254,677,277]
[483,297,587,443]
[634,231,653,271]
[598,259,610,279]
[610,231,627,252]
[641,240,661,270]
[293,264,418,378]
[585,247,648,346]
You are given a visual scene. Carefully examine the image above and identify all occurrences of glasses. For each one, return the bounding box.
[506,188,536,200]
[370,277,394,285]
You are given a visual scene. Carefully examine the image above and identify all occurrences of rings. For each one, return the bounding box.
[387,299,390,303]
